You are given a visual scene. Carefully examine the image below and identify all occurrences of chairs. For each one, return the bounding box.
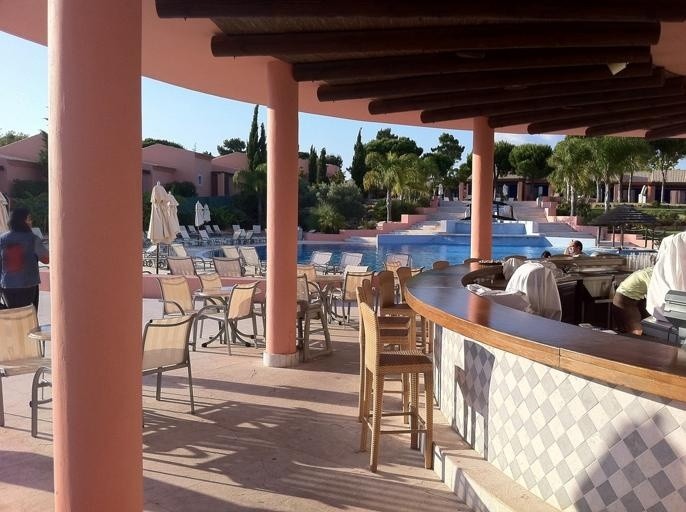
[336,251,527,471]
[442,193,516,204]
[141,223,332,416]
[0,306,48,426]
[27,321,52,437]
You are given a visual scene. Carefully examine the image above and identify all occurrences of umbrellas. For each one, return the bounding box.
[146,179,178,245]
[167,190,181,237]
[203,203,212,224]
[0,191,10,235]
[193,200,205,227]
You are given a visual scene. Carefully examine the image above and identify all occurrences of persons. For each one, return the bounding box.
[438,183,444,199]
[612,261,656,338]
[0,206,49,316]
[563,239,588,259]
[539,249,552,259]
[502,183,509,200]
